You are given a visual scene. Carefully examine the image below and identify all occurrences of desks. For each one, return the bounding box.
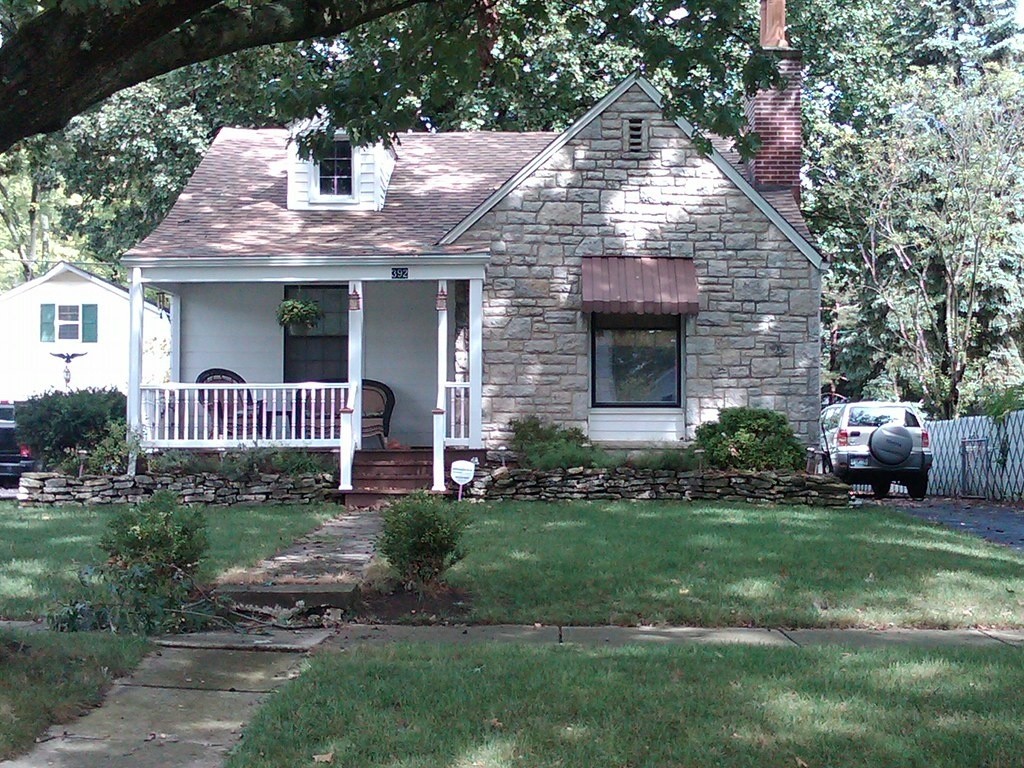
[250,409,294,440]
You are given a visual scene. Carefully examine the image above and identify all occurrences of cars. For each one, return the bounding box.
[0,399,45,483]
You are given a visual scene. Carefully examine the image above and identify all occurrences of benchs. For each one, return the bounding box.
[294,377,395,450]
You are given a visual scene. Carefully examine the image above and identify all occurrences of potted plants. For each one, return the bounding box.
[275,297,328,337]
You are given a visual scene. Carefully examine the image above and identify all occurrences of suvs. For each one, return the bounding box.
[817,401,933,501]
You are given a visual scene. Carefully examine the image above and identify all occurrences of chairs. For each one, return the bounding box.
[196,368,266,439]
